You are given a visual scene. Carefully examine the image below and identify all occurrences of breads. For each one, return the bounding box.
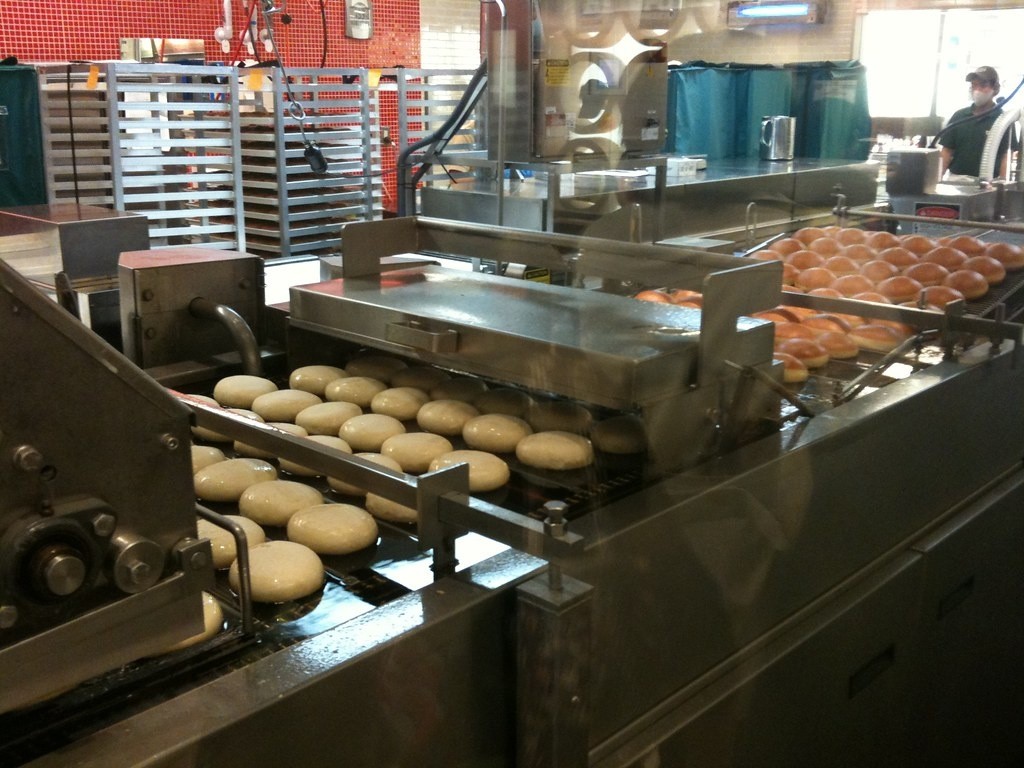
[635,226,1024,383]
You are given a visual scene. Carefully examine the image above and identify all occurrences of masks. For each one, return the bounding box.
[973,77,1000,107]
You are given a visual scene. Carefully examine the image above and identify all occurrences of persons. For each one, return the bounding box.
[938,65,1020,181]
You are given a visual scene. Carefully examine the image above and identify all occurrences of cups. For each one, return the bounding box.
[760,116,796,160]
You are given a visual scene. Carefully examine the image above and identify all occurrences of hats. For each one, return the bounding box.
[966,66,998,81]
